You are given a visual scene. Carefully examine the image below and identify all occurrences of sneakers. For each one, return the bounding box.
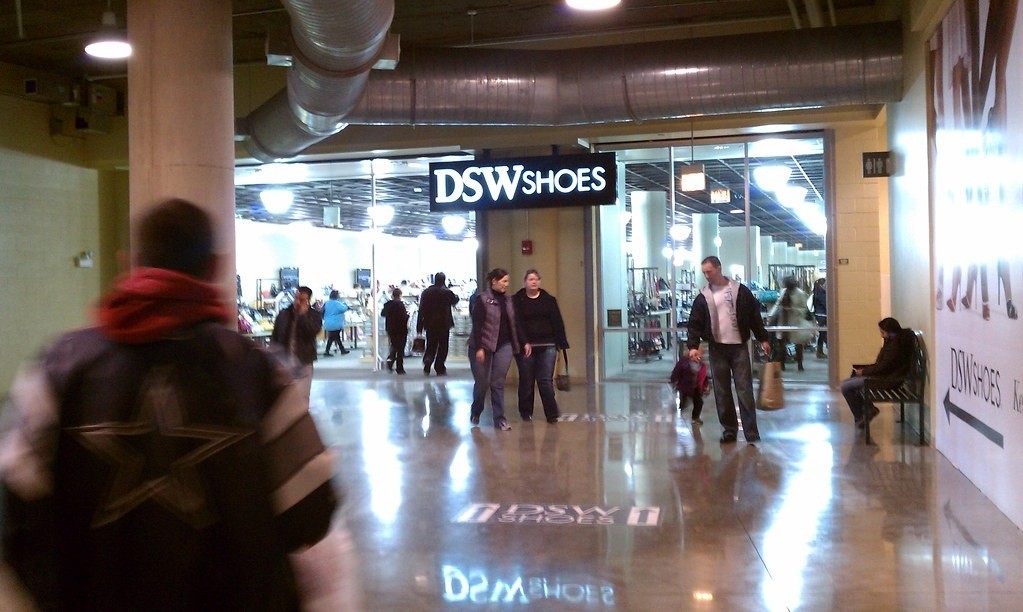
[470,412,479,425]
[692,417,703,425]
[495,419,513,431]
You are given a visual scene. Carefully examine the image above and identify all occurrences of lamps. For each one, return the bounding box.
[710,160,731,204]
[679,119,704,191]
[85,2,134,59]
[77,251,95,269]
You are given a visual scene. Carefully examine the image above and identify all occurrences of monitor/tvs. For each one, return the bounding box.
[356,269,371,289]
[280,267,299,291]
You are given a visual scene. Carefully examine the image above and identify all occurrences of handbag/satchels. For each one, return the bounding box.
[321,303,325,319]
[412,333,424,352]
[756,353,784,410]
[556,347,571,391]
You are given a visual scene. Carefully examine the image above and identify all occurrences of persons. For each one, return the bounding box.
[0,198,362,612]
[767,275,814,371]
[669,346,711,426]
[267,287,322,409]
[381,288,409,374]
[841,317,914,427]
[321,291,350,357]
[812,277,829,358]
[417,272,459,375]
[469,288,477,316]
[686,257,770,443]
[467,269,533,430]
[933,260,1018,321]
[511,268,568,423]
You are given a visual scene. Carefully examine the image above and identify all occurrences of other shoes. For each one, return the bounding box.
[341,349,350,354]
[547,416,558,424]
[386,360,405,374]
[424,359,447,376]
[858,407,879,427]
[324,350,333,357]
[744,430,760,442]
[523,414,532,421]
[723,429,736,441]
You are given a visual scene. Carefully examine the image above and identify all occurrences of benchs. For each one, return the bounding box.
[851,326,928,446]
